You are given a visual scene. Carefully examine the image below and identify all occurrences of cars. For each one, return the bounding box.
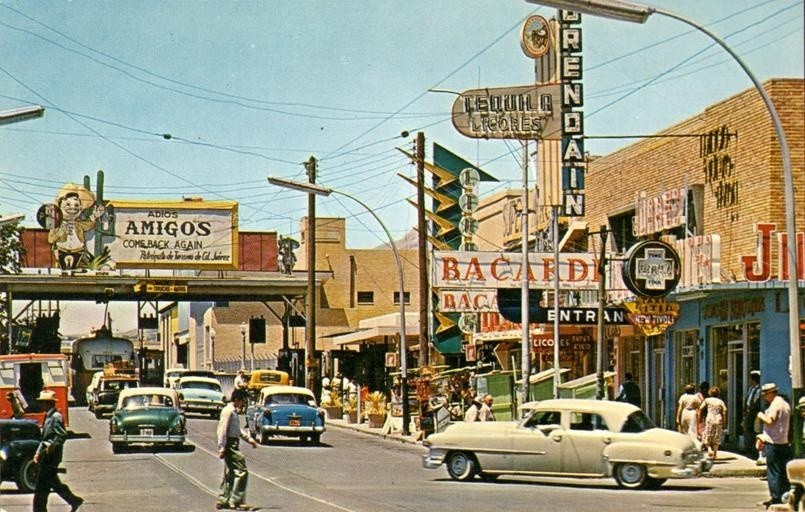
[82,357,326,447]
[422,397,703,488]
[0,418,68,494]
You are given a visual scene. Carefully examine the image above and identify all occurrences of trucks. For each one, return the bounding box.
[0,350,74,440]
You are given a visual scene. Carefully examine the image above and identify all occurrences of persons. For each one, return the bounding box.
[4,391,25,420]
[478,394,496,422]
[614,372,642,409]
[233,371,248,390]
[214,387,262,510]
[31,390,84,512]
[321,367,370,405]
[677,367,805,506]
[464,396,483,422]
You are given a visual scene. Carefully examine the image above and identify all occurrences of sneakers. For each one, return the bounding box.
[228,501,255,511]
[215,499,230,510]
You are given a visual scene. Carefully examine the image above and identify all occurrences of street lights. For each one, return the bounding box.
[527,0,805,509]
[0,104,43,135]
[588,219,619,399]
[267,172,410,436]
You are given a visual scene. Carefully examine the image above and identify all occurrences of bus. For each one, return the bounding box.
[63,333,136,408]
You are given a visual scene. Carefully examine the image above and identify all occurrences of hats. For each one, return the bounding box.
[35,389,61,403]
[760,382,780,395]
[474,396,483,406]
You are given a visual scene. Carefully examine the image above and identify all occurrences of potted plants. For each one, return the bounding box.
[320,384,342,419]
[343,396,365,423]
[365,391,385,428]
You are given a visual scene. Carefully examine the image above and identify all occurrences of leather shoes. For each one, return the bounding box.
[763,498,780,505]
[68,496,84,512]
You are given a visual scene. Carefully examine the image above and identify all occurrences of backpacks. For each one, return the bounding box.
[623,384,641,405]
